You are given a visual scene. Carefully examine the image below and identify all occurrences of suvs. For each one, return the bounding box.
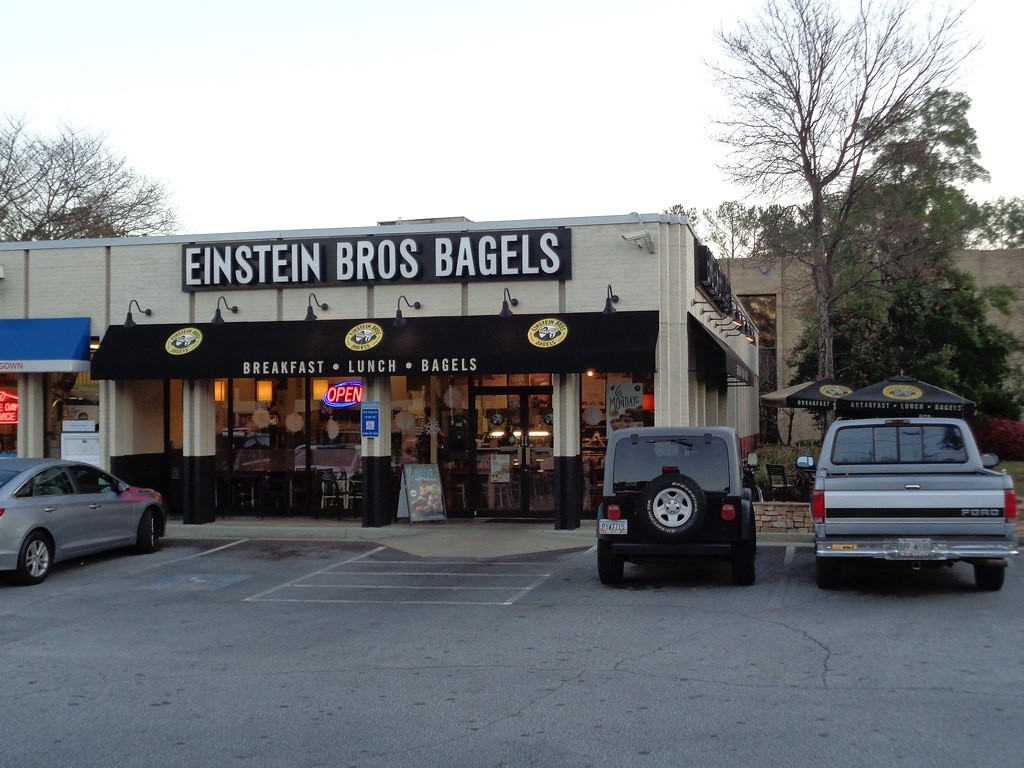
[595,430,759,586]
[219,428,270,474]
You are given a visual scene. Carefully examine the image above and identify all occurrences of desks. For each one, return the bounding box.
[803,469,817,502]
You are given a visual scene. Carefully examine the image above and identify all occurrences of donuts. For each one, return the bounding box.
[411,484,444,514]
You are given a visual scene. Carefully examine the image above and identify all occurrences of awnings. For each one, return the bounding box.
[90,309,660,381]
[0,317,92,373]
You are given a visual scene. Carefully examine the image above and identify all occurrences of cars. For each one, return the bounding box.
[0,456,169,582]
[288,443,419,478]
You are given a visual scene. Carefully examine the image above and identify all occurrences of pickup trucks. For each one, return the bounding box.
[794,416,1022,589]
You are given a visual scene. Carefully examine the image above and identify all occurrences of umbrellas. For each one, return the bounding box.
[759,378,861,439]
[836,375,976,413]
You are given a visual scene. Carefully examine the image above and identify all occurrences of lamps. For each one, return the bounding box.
[498,288,519,318]
[211,296,238,325]
[122,300,152,329]
[394,296,421,328]
[304,293,328,322]
[601,284,619,314]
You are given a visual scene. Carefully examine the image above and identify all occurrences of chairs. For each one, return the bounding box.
[767,464,795,501]
[37,473,63,495]
